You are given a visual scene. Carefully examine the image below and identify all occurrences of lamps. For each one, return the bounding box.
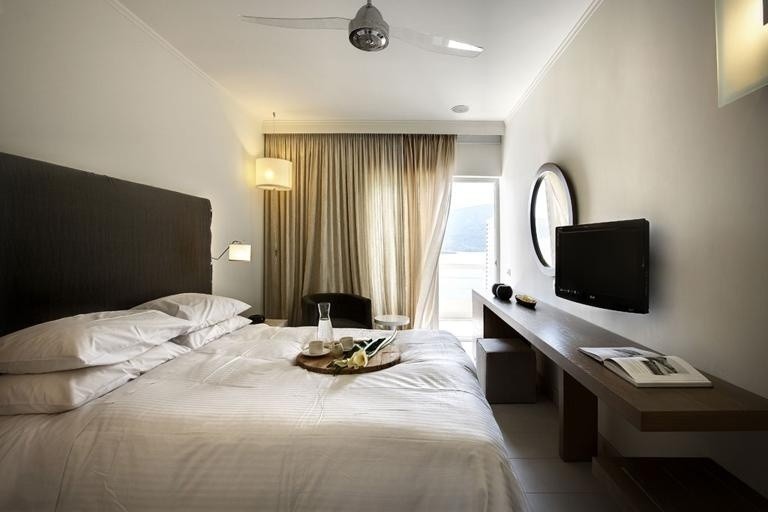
[255,156,294,263]
[210,240,252,264]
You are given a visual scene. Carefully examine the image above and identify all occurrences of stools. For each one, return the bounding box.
[374,314,410,330]
[476,338,537,405]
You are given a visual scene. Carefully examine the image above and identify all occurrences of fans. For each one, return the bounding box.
[238,0,484,60]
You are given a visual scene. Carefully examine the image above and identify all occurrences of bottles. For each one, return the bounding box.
[316,302,334,342]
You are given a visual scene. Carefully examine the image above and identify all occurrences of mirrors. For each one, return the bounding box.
[527,162,575,277]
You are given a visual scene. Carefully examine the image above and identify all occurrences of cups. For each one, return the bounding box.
[340,337,353,349]
[308,342,323,354]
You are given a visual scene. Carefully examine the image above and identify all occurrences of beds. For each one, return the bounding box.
[0,152,530,512]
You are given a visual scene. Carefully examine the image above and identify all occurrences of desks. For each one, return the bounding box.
[471,288,768,510]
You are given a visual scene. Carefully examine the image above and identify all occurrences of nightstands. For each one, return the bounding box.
[248,318,289,327]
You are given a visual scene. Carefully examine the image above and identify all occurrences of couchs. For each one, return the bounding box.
[301,293,373,329]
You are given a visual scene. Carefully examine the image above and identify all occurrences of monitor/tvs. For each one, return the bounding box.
[554,218,650,314]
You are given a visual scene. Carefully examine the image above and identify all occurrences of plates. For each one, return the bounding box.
[302,349,330,356]
[515,295,538,306]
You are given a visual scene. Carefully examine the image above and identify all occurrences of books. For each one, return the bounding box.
[577,347,714,390]
